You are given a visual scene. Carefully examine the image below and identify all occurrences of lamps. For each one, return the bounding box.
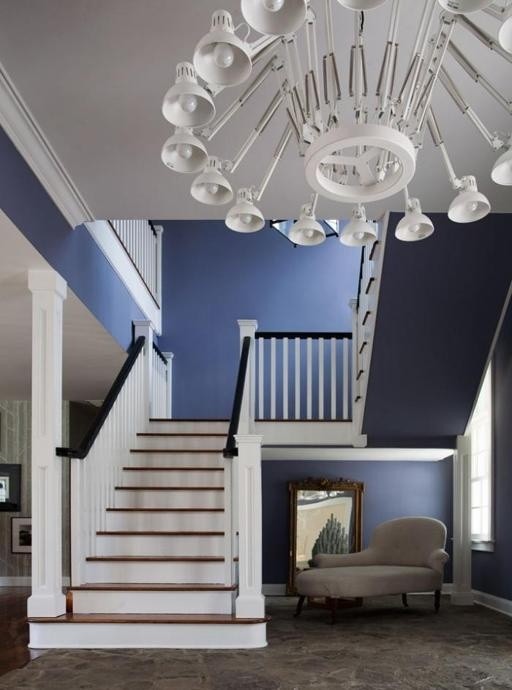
[161,0,511,247]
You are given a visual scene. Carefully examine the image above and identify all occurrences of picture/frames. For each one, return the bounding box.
[0,463,22,513]
[11,517,31,554]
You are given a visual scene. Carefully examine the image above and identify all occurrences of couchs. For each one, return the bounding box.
[293,516,450,625]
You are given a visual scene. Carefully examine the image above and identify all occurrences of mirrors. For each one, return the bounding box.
[287,476,365,593]
